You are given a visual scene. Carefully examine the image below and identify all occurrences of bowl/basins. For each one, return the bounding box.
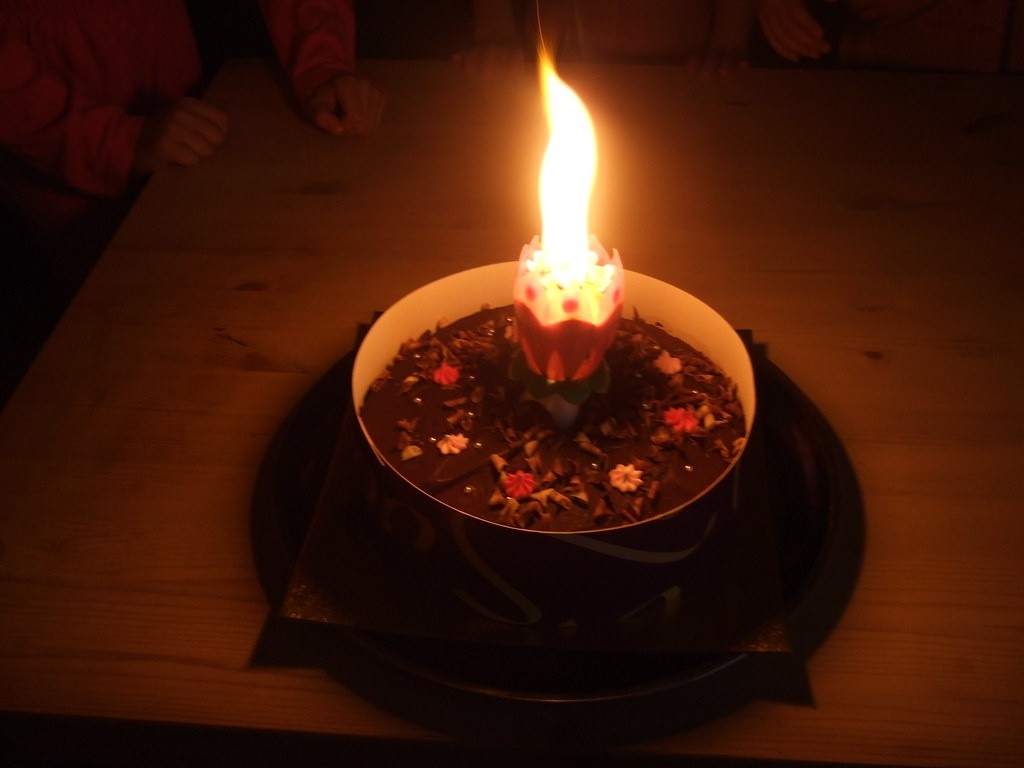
[351,262,758,534]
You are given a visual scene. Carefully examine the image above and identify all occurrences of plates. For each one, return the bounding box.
[249,351,866,749]
[280,314,793,655]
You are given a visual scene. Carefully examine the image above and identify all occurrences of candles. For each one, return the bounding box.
[514,62,628,384]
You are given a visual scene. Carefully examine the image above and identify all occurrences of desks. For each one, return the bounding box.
[0,57,1024,768]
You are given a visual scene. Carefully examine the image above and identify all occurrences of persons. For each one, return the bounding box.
[452,0,1024,80]
[0,0,386,294]
